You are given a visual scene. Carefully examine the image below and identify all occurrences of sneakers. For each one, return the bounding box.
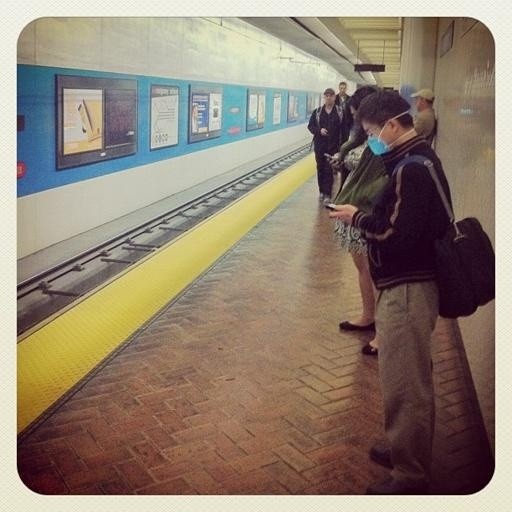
[319,192,332,206]
[367,474,429,495]
[370,444,393,468]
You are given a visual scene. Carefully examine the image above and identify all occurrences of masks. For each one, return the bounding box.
[367,122,391,156]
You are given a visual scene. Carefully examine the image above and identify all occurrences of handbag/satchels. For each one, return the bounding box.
[433,218,495,318]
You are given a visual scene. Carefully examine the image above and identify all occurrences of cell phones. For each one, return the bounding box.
[325,204,336,210]
[323,153,332,159]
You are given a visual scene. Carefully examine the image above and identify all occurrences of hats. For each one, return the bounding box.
[324,89,335,95]
[410,89,434,102]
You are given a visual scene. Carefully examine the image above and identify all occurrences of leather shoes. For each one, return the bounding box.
[362,342,378,355]
[339,320,376,331]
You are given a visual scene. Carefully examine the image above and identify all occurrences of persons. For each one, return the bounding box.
[328,88,453,495]
[307,81,436,356]
